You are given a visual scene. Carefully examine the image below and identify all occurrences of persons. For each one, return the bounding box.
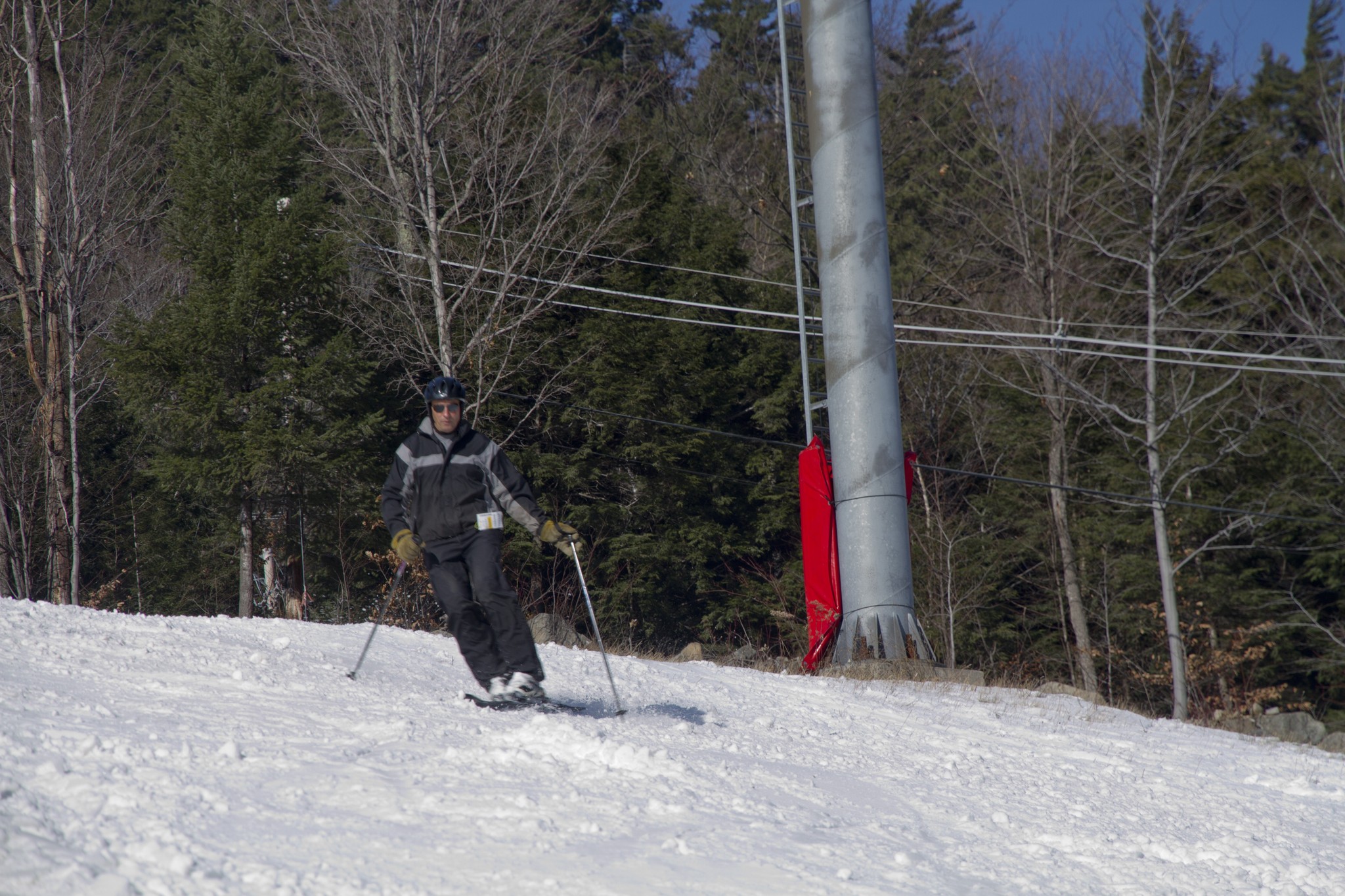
[381,377,583,702]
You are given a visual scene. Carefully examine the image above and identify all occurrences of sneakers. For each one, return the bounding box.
[487,670,545,702]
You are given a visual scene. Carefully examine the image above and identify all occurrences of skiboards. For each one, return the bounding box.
[462,693,625,720]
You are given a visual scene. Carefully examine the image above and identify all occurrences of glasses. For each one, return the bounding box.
[431,402,461,412]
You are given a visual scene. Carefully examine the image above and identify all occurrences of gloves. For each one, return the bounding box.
[390,529,426,562]
[538,521,582,556]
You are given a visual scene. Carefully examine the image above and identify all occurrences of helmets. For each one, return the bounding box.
[424,376,466,418]
